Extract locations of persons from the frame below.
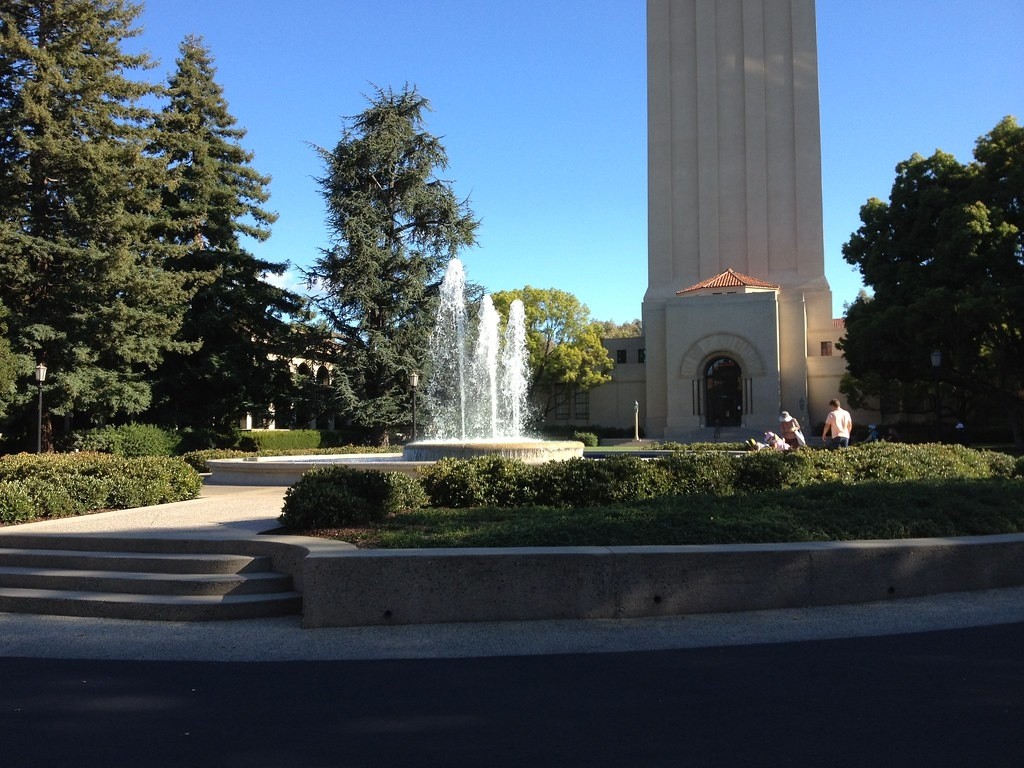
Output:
[747,432,790,453]
[778,411,806,450]
[822,399,852,448]
[887,427,898,442]
[864,425,879,443]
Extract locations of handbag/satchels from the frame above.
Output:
[792,418,806,446]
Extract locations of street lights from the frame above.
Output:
[633,400,642,442]
[33,362,48,456]
[408,372,419,441]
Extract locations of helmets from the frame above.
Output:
[868,425,876,430]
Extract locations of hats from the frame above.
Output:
[779,411,792,422]
